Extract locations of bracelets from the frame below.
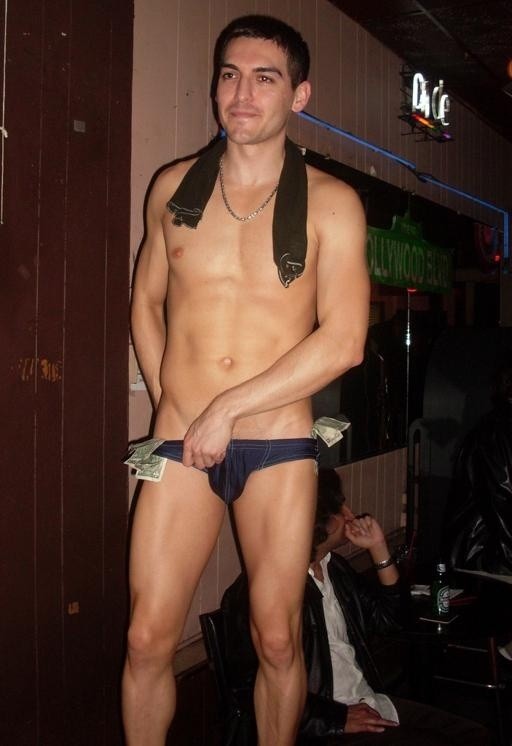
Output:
[374,557,396,571]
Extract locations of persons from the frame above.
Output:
[112,10,372,746]
[216,457,505,745]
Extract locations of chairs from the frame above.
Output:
[198,601,250,743]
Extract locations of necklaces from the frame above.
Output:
[217,151,281,222]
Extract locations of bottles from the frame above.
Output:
[433,557,450,615]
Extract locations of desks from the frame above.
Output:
[369,572,510,741]
